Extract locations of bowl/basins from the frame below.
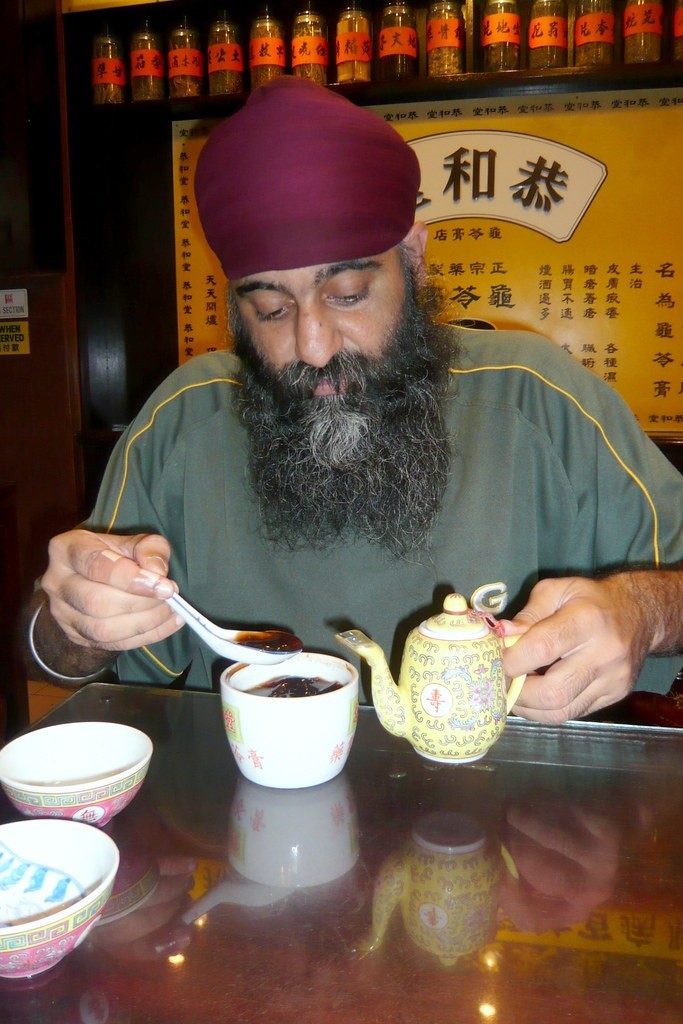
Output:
[0,818,120,979]
[226,775,361,889]
[97,816,158,927]
[0,722,153,831]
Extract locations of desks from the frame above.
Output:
[0,681,683,1024]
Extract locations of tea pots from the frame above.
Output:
[358,814,515,969]
[335,581,530,764]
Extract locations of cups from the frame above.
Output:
[220,653,360,790]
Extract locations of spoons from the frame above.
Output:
[182,879,287,926]
[157,586,301,664]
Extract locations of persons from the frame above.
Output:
[77,784,683,945]
[16,78,682,727]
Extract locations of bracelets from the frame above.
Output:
[28,599,117,685]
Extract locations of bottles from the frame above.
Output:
[92,0,683,106]
